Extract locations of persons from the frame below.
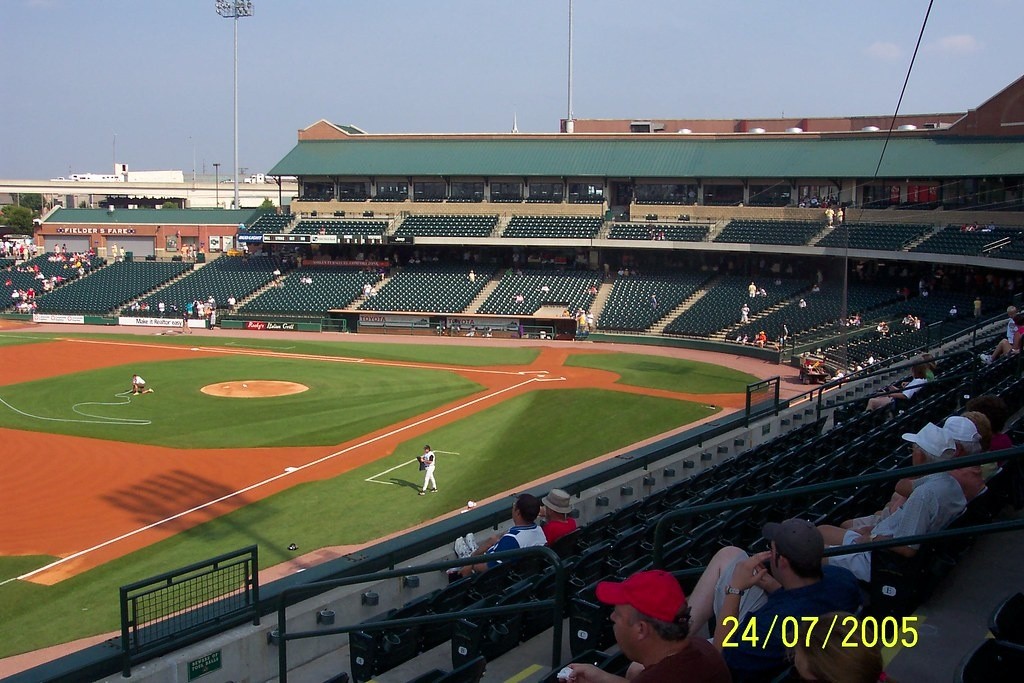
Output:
[447,489,577,576]
[832,354,875,381]
[273,226,386,301]
[888,354,936,395]
[0,221,249,333]
[504,251,608,338]
[409,253,493,337]
[867,364,927,410]
[562,397,1013,683]
[647,223,665,240]
[959,221,999,234]
[417,445,438,495]
[618,267,657,310]
[132,374,154,395]
[796,195,843,228]
[734,266,1024,364]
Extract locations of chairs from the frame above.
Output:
[392,217,1023,654]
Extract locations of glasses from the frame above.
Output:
[765,542,780,556]
[907,443,921,454]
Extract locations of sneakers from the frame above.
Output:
[454,533,480,559]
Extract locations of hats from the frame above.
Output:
[596,570,693,624]
[762,519,824,566]
[541,489,573,513]
[759,331,764,334]
[422,445,429,449]
[800,299,804,302]
[943,416,980,442]
[901,421,956,459]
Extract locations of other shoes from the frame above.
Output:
[826,224,834,228]
[133,392,139,396]
[429,487,438,493]
[418,491,425,496]
[980,353,993,365]
[149,388,154,393]
